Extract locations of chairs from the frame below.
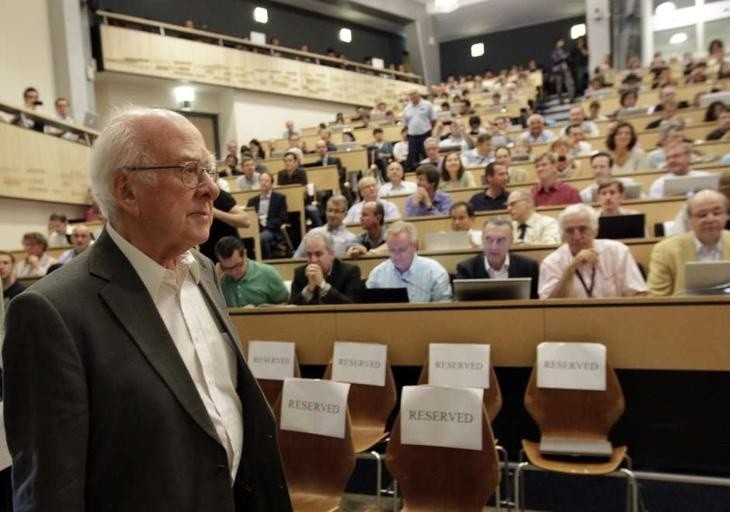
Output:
[387,341,510,512]
[244,339,301,412]
[514,341,637,512]
[271,377,355,512]
[323,342,397,512]
[385,385,503,512]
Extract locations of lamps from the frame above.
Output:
[173,82,193,102]
[655,0,675,19]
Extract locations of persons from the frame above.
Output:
[54,224,96,264]
[202,184,249,263]
[237,160,271,189]
[558,107,600,140]
[591,182,653,238]
[370,128,394,181]
[650,141,716,198]
[653,84,690,112]
[588,101,608,120]
[433,56,543,117]
[539,205,647,297]
[294,194,358,260]
[645,101,687,130]
[545,141,581,176]
[342,201,391,256]
[0,251,29,307]
[214,237,290,308]
[382,160,416,196]
[514,114,556,147]
[401,163,453,219]
[704,101,726,120]
[284,107,366,155]
[586,39,729,92]
[278,151,322,227]
[247,169,289,260]
[648,189,730,296]
[16,87,46,129]
[177,17,427,78]
[717,110,729,127]
[468,116,514,148]
[461,133,497,167]
[0,109,296,512]
[580,152,640,203]
[647,126,696,168]
[605,123,646,172]
[706,116,730,142]
[402,88,436,158]
[456,217,538,298]
[15,233,58,278]
[608,91,638,119]
[437,153,476,187]
[289,232,366,303]
[223,139,286,161]
[394,127,409,171]
[435,108,604,149]
[575,35,589,100]
[221,155,241,179]
[421,138,445,167]
[432,115,477,152]
[50,211,72,248]
[466,159,512,211]
[344,175,401,225]
[476,142,535,188]
[501,189,563,245]
[312,140,353,225]
[440,200,483,249]
[48,93,80,138]
[357,91,414,129]
[530,152,582,206]
[553,40,574,105]
[366,221,455,303]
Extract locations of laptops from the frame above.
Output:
[662,175,720,197]
[699,92,730,108]
[597,214,644,239]
[684,261,729,294]
[451,278,532,301]
[591,185,641,202]
[352,289,410,303]
[423,232,472,253]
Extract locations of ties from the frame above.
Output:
[518,223,529,239]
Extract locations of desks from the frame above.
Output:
[504,102,709,134]
[216,239,670,283]
[224,165,342,222]
[377,164,730,214]
[223,295,730,373]
[581,79,730,115]
[405,140,730,182]
[228,181,306,239]
[17,275,43,288]
[261,124,406,155]
[445,122,725,148]
[81,209,261,264]
[3,246,75,261]
[286,197,686,237]
[368,68,505,120]
[502,66,550,115]
[217,148,368,179]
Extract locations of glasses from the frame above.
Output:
[327,208,345,213]
[363,184,377,189]
[667,152,686,159]
[220,256,244,271]
[507,198,529,206]
[123,162,219,188]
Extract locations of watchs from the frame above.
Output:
[316,277,331,293]
